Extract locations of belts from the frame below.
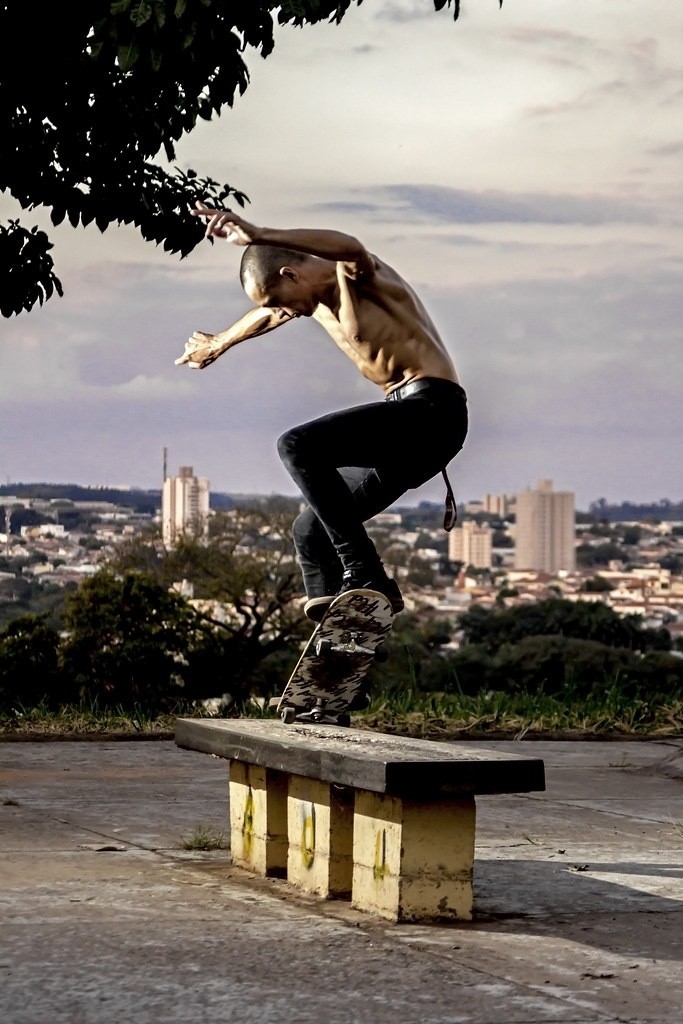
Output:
[385,378,467,401]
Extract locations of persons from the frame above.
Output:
[173,200,469,711]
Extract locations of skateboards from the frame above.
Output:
[277,587,396,726]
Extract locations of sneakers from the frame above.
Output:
[304,570,404,622]
[270,676,371,711]
